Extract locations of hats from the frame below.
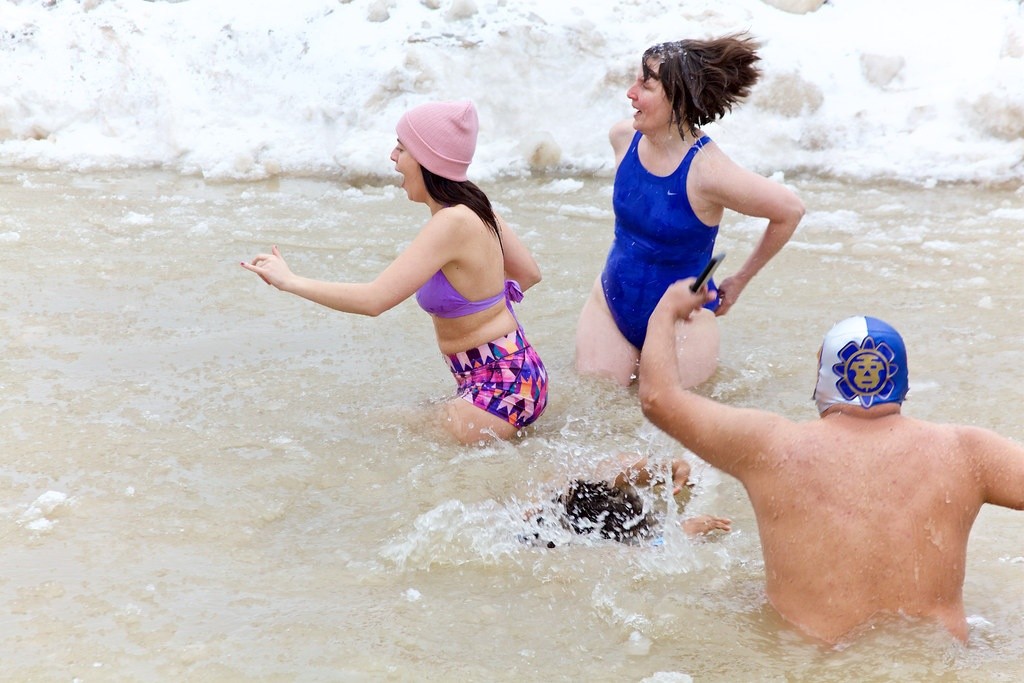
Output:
[813,315,911,411]
[395,103,478,182]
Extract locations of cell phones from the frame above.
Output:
[690,252,726,292]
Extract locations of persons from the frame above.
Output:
[239,97,551,447]
[571,29,809,390]
[520,453,733,550]
[636,274,1023,649]
